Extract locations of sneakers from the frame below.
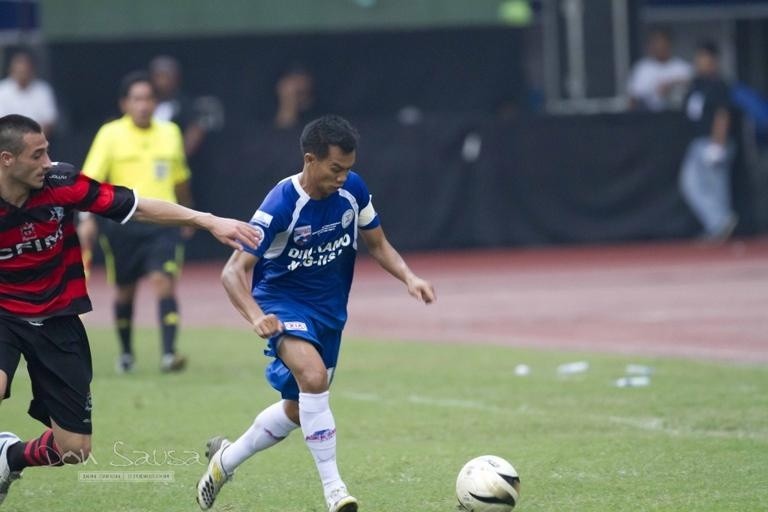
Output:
[115,351,186,375]
[196,436,233,512]
[0,431,25,504]
[328,497,359,512]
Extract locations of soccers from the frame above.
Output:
[455,455,520,512]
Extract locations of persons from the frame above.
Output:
[679,34,747,247]
[0,113,264,505]
[258,69,313,158]
[623,22,688,114]
[147,53,225,162]
[193,114,436,512]
[76,68,198,372]
[1,42,62,141]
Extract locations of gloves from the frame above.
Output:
[702,143,727,169]
